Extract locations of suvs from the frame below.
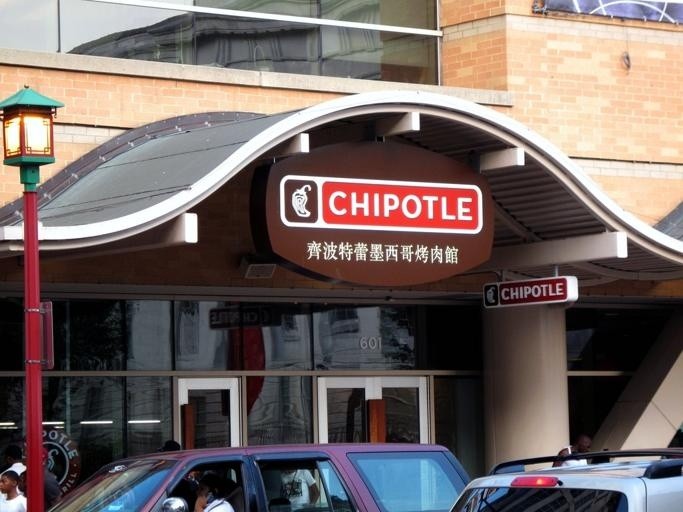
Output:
[448,446,681,510]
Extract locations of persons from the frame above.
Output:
[192,465,238,512]
[278,468,321,511]
[0,444,27,477]
[21,448,62,512]
[551,432,595,469]
[0,470,27,512]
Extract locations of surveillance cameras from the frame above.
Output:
[375,134,386,142]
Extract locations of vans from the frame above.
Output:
[46,443,472,512]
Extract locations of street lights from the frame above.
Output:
[0,86,64,512]
